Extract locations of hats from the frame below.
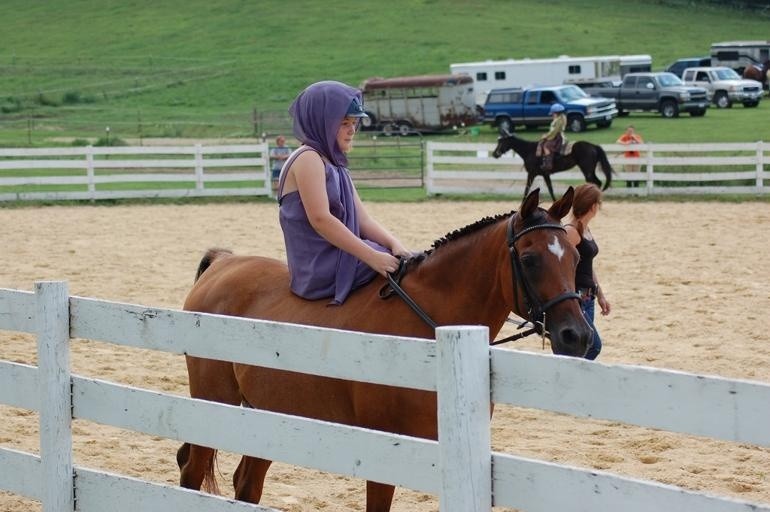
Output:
[344,95,369,121]
[547,102,566,115]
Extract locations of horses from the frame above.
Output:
[491,127,618,207]
[175,185,597,512]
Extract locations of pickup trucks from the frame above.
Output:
[482,57,765,137]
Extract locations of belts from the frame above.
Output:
[579,286,594,297]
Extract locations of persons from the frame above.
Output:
[616,127,646,188]
[540,104,568,175]
[278,81,416,307]
[267,134,293,191]
